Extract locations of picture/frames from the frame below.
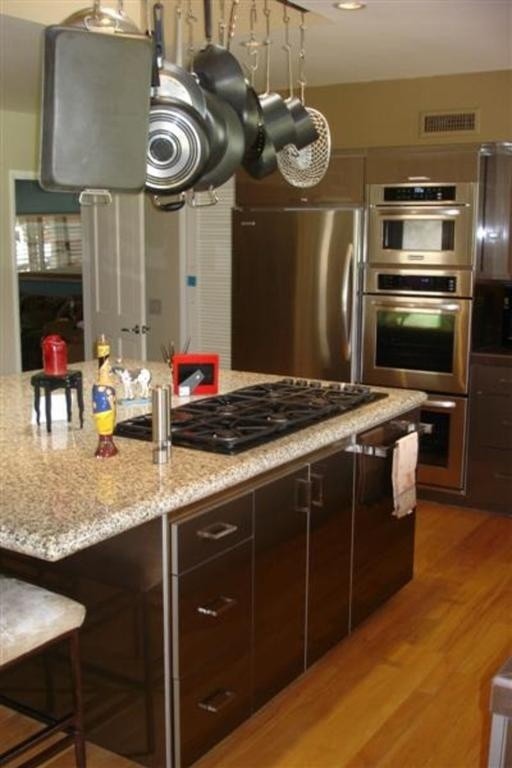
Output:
[170,352,219,398]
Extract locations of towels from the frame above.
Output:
[391,433,418,520]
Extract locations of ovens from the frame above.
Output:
[360,180,477,495]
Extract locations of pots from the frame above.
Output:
[60,0,320,213]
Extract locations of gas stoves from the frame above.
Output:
[114,376,390,457]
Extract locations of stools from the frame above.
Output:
[0,572,89,765]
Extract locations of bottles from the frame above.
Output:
[91,332,120,459]
[147,383,175,455]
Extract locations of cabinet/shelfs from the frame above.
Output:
[355,404,417,631]
[253,441,352,712]
[169,491,255,768]
[469,358,510,511]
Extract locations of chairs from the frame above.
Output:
[16,288,81,372]
